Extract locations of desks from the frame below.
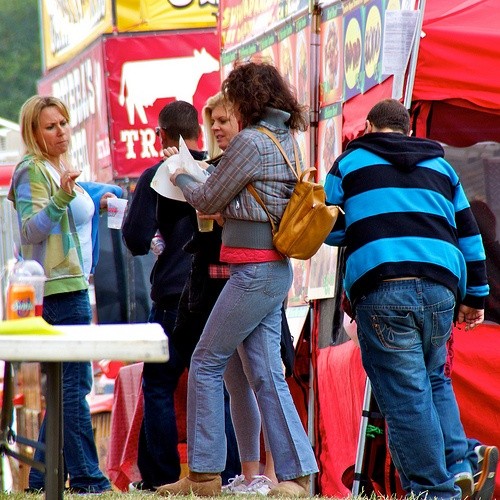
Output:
[0,322,169,500]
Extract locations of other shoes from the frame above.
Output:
[156,469,222,496]
[266,475,310,498]
[128,477,157,491]
[471,445,498,500]
[449,459,474,498]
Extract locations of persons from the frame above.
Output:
[320,99,499,500]
[123,54,320,496]
[7,95,123,493]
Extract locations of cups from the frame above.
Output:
[152,232,165,255]
[195,209,214,233]
[106,197,128,229]
[8,260,47,316]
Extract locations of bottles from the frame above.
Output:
[6,267,36,320]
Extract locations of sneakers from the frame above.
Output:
[232,474,274,496]
[222,475,251,493]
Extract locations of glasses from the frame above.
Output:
[155,127,166,135]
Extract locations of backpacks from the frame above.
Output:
[244,128,344,260]
